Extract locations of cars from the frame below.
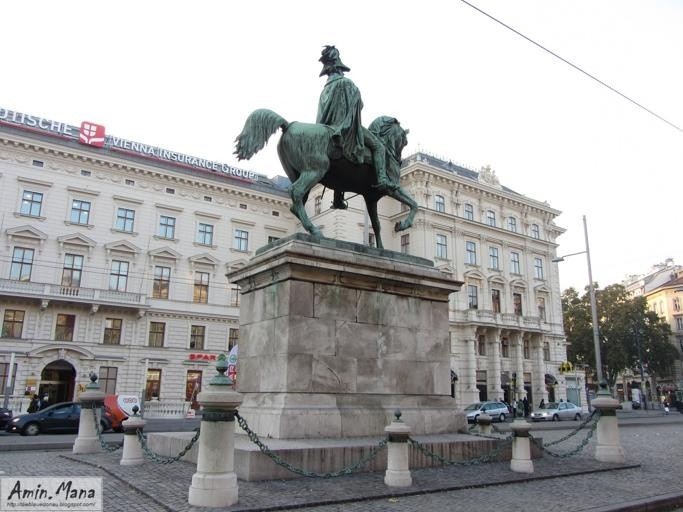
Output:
[462,400,509,423]
[616,401,639,410]
[0,408,13,428]
[529,401,582,422]
[5,402,111,435]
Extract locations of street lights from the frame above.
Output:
[550,215,604,394]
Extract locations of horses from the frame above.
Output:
[232,108,418,251]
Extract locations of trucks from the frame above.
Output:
[102,394,141,432]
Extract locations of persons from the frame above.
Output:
[28,394,38,413]
[540,399,544,409]
[318,46,400,208]
[499,397,529,418]
[21,391,32,413]
[40,395,49,410]
[663,399,669,416]
